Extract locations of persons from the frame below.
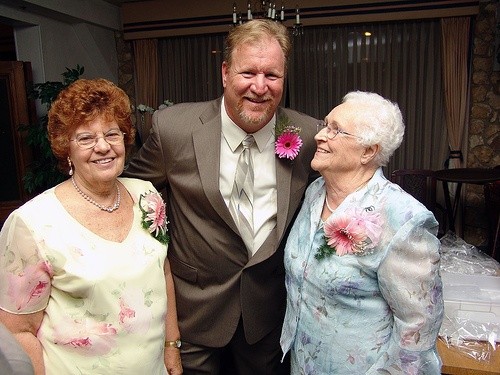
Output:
[119,19,330,375]
[280,90,443,375]
[0,76,183,375]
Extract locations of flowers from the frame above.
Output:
[132,97,175,112]
[314,203,384,260]
[139,190,169,244]
[271,113,303,160]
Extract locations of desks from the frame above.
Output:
[433,168,500,258]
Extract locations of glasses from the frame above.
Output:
[68,128,126,150]
[316,120,361,140]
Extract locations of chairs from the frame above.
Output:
[391,169,446,238]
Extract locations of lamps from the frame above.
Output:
[233,0,304,36]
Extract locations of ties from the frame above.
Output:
[228,135,255,261]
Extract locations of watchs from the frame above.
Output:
[164,337,182,349]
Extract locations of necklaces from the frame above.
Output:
[71,172,122,212]
